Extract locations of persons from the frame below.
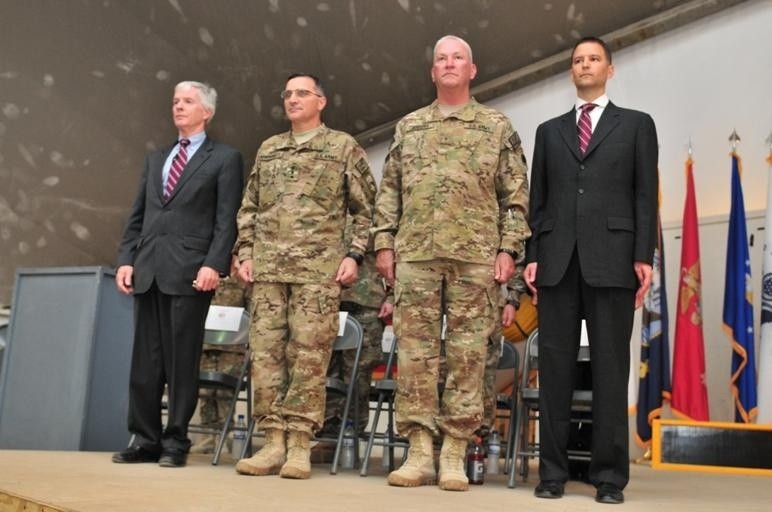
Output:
[190,268,254,454]
[521,37,659,503]
[232,75,377,480]
[370,35,532,492]
[112,81,243,467]
[434,266,525,472]
[310,235,395,470]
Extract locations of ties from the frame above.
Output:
[164,139,189,200]
[578,103,597,152]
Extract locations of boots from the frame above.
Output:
[281,430,314,479]
[439,433,469,491]
[309,441,336,464]
[235,427,287,476]
[188,430,217,454]
[216,432,231,457]
[387,430,437,488]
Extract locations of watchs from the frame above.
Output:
[344,252,363,266]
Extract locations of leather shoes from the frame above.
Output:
[159,446,187,467]
[596,482,624,505]
[533,478,566,498]
[112,438,165,463]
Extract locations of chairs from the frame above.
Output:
[509,324,592,488]
[361,329,520,483]
[162,303,255,464]
[240,311,359,472]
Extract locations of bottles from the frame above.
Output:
[230,413,248,460]
[379,425,397,466]
[486,429,502,476]
[466,435,485,486]
[342,419,356,470]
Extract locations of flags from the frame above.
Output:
[634,183,671,450]
[757,155,772,424]
[671,158,709,422]
[722,151,759,422]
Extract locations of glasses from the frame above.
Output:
[280,90,321,98]
